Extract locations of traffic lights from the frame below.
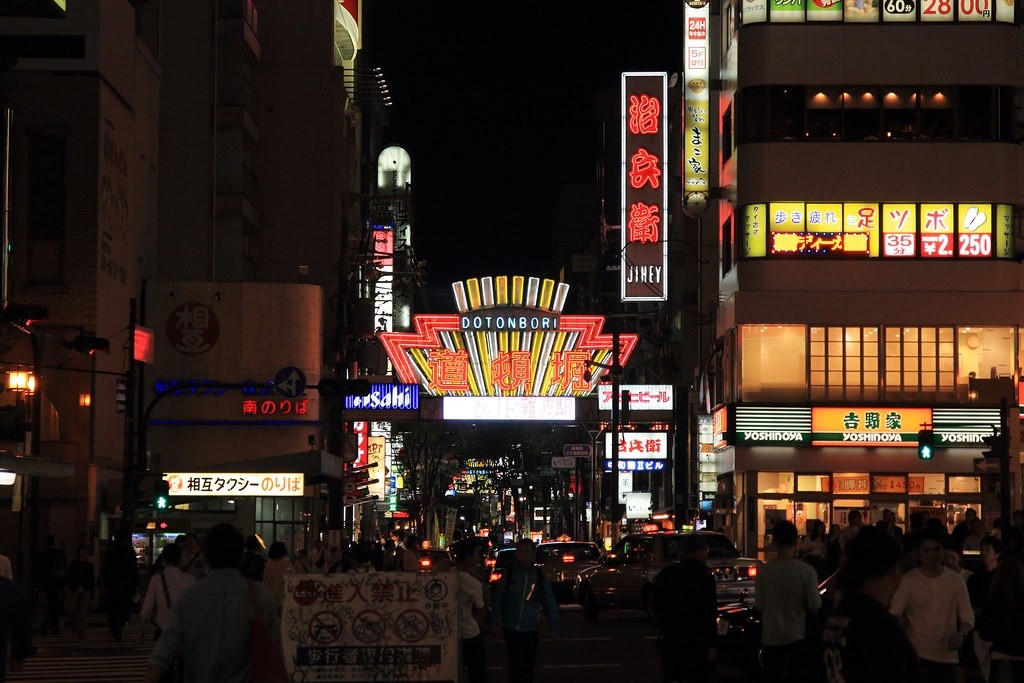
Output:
[154,480,169,511]
[74,334,111,356]
[918,430,935,459]
[158,522,168,528]
[982,437,1001,462]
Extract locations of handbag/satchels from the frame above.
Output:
[248,577,290,683]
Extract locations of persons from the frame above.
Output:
[755,520,822,682]
[0,523,491,683]
[801,508,1024,682]
[491,538,560,683]
[651,533,718,682]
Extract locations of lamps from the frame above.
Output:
[299,266,310,283]
[214,293,220,301]
[309,435,315,451]
[414,261,427,288]
[169,292,176,300]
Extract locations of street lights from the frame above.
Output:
[582,363,619,553]
[6,364,39,580]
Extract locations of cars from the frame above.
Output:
[418,538,609,603]
[571,530,763,626]
